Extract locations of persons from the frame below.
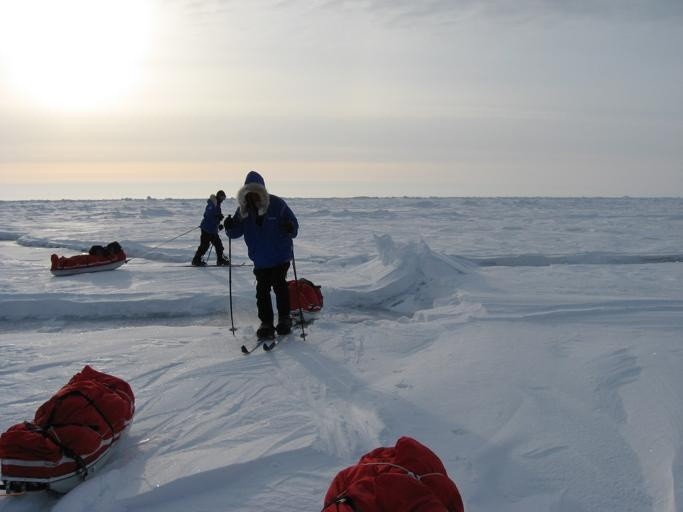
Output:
[223,171,297,339]
[189,190,229,265]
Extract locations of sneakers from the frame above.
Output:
[191,257,230,266]
[254,317,291,338]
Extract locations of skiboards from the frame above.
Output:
[165,262,255,267]
[242,326,298,354]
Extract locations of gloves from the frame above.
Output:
[223,214,234,230]
[281,218,294,234]
